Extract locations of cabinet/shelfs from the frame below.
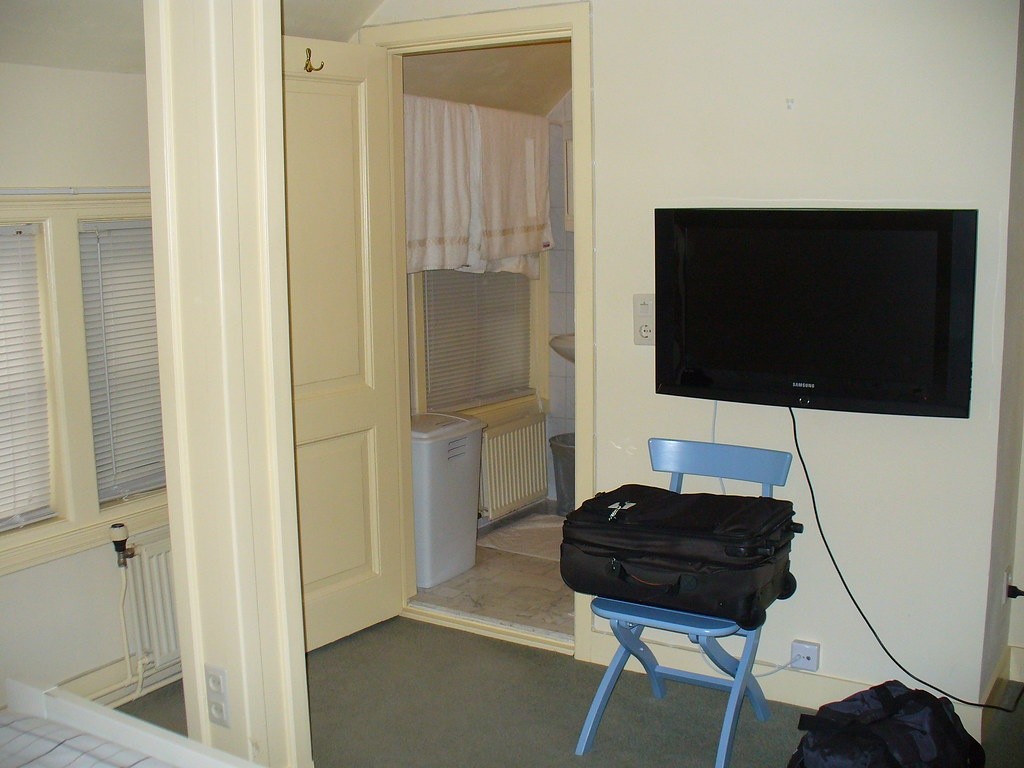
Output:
[457,394,551,520]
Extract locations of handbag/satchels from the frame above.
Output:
[792,677,985,768]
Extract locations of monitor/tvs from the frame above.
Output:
[655,207,979,420]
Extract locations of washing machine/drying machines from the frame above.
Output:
[410,410,488,589]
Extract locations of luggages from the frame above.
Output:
[562,482,802,630]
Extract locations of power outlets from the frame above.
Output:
[632,294,655,347]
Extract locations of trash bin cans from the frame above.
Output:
[549,433,575,517]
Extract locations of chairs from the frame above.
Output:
[569,436,794,768]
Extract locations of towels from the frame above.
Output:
[404,92,556,280]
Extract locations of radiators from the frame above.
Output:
[126,539,184,674]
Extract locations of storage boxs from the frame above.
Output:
[411,410,490,590]
